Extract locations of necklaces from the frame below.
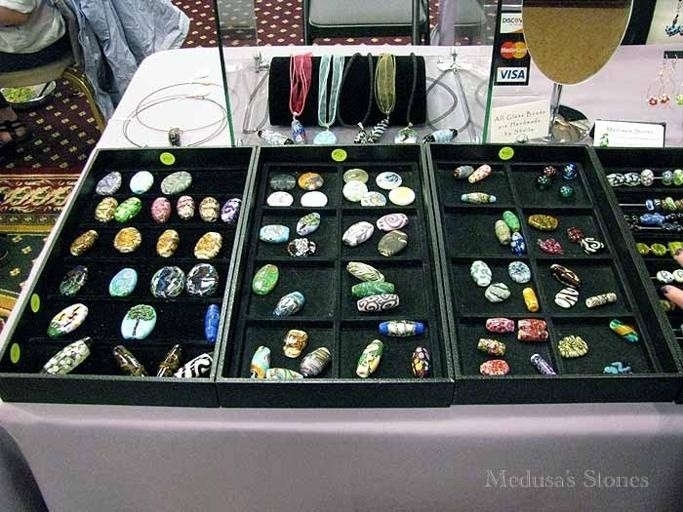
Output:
[241,50,471,148]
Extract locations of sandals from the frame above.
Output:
[0,118,30,148]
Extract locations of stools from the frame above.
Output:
[0,55,106,139]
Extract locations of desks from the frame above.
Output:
[0,46,682,511]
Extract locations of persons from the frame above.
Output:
[0,0,70,150]
[660,247,682,312]
